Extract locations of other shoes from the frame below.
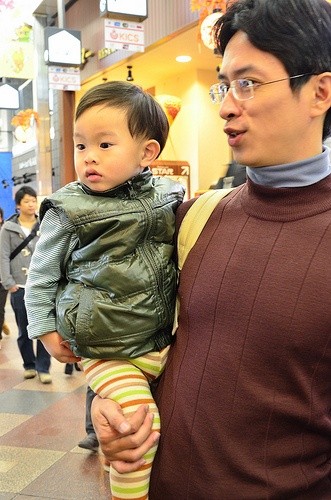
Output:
[3,324,9,335]
[23,369,36,378]
[74,362,81,371]
[65,363,73,374]
[39,373,52,383]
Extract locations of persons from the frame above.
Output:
[23,0,330,500]
[0,187,85,384]
[25,80,186,500]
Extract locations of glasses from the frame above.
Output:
[208,73,319,104]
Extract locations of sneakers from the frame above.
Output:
[78,433,99,452]
